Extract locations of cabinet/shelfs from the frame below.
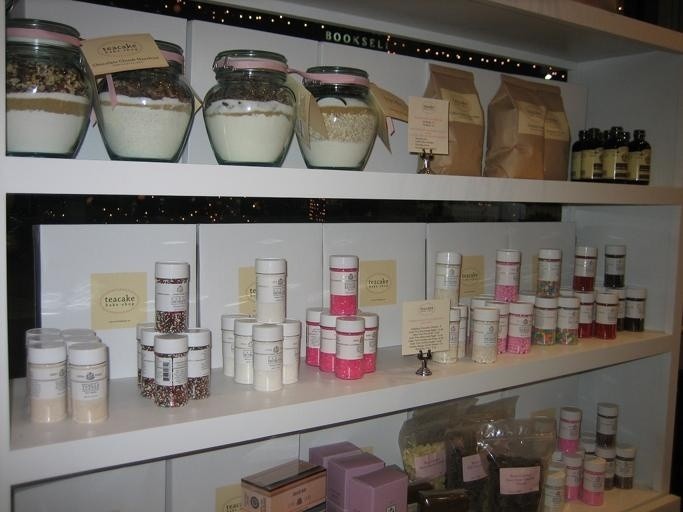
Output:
[1,0,683,512]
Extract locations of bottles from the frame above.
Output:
[495,247,519,303]
[27,325,111,423]
[93,39,196,163]
[532,292,647,348]
[328,255,360,316]
[543,432,635,512]
[256,258,289,324]
[596,401,619,449]
[220,313,302,392]
[433,251,463,310]
[304,308,380,381]
[6,15,93,156]
[602,244,626,290]
[152,262,189,333]
[201,50,299,166]
[561,406,583,454]
[431,292,536,364]
[536,247,561,298]
[572,245,597,292]
[136,321,212,410]
[298,67,376,170]
[572,127,654,185]
